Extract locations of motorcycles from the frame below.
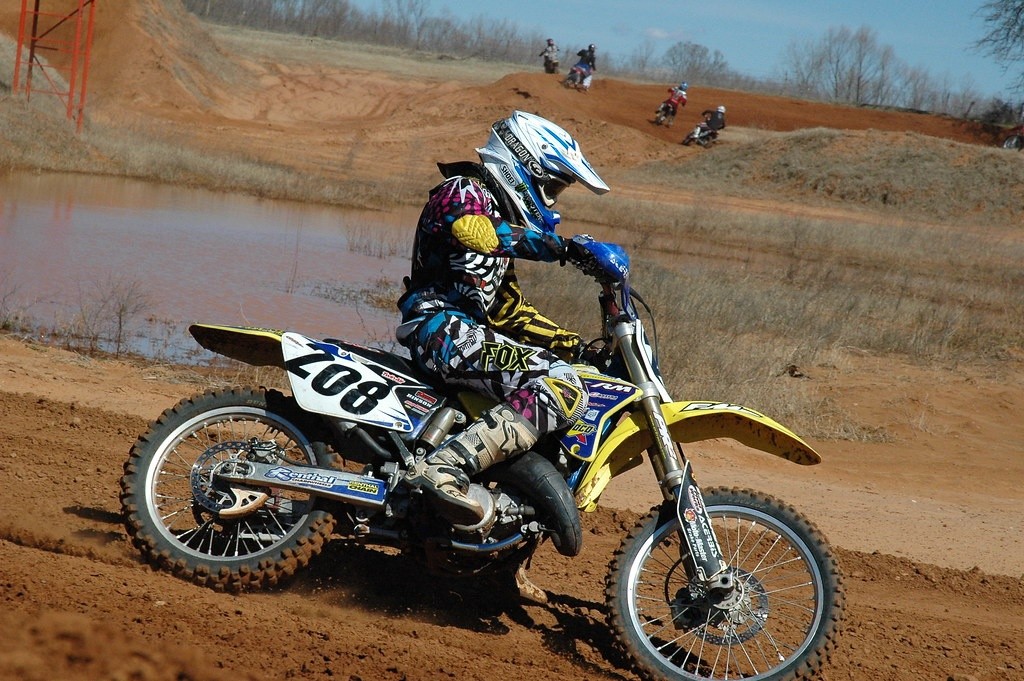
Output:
[565,64,588,88]
[119,248,846,681]
[657,103,674,124]
[681,116,718,148]
[545,60,561,76]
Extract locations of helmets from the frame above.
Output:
[680,83,687,91]
[717,105,726,114]
[589,44,596,50]
[547,38,553,44]
[474,110,610,234]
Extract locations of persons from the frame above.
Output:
[656,79,687,124]
[691,105,725,138]
[559,43,598,89]
[540,37,561,67]
[398,110,629,605]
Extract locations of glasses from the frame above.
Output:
[536,172,569,207]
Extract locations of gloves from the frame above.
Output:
[565,232,605,273]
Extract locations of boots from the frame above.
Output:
[488,548,549,604]
[387,403,540,524]
